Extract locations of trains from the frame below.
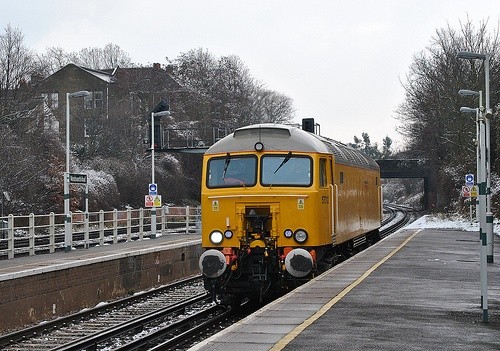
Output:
[198,118,384,304]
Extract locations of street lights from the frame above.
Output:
[459,104,481,222]
[63,89,91,255]
[150,111,171,236]
[456,49,495,263]
[457,89,487,253]
